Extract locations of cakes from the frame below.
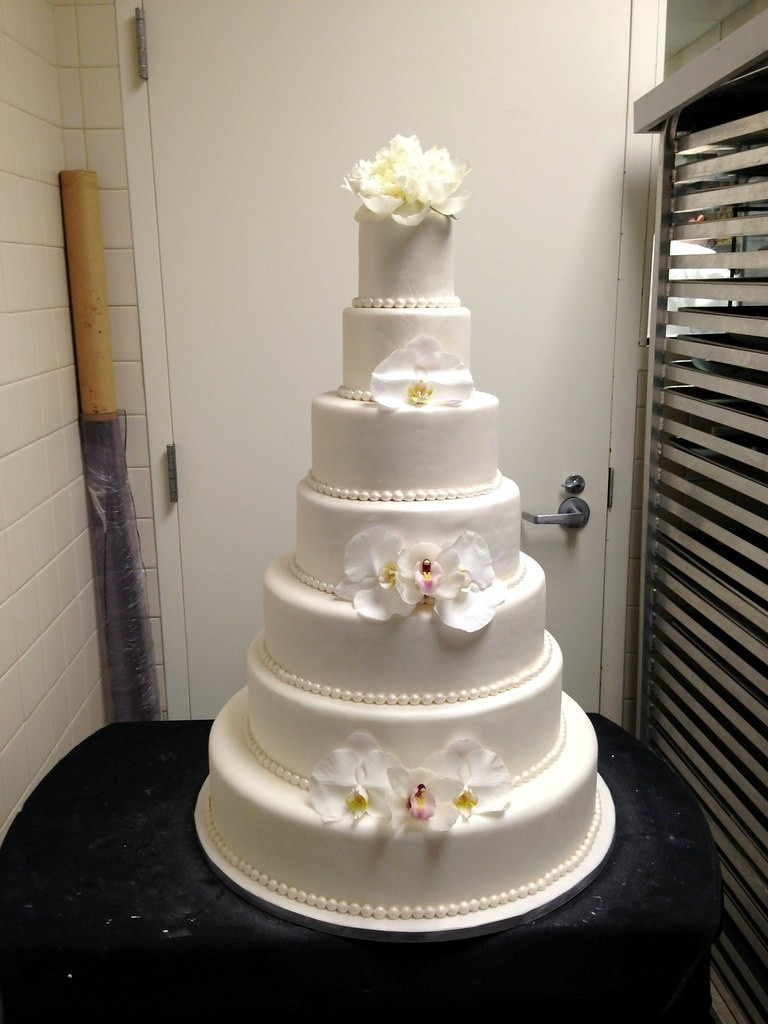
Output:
[205,132,601,921]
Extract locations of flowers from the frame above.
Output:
[338,131,472,226]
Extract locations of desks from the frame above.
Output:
[0,711,727,1024]
